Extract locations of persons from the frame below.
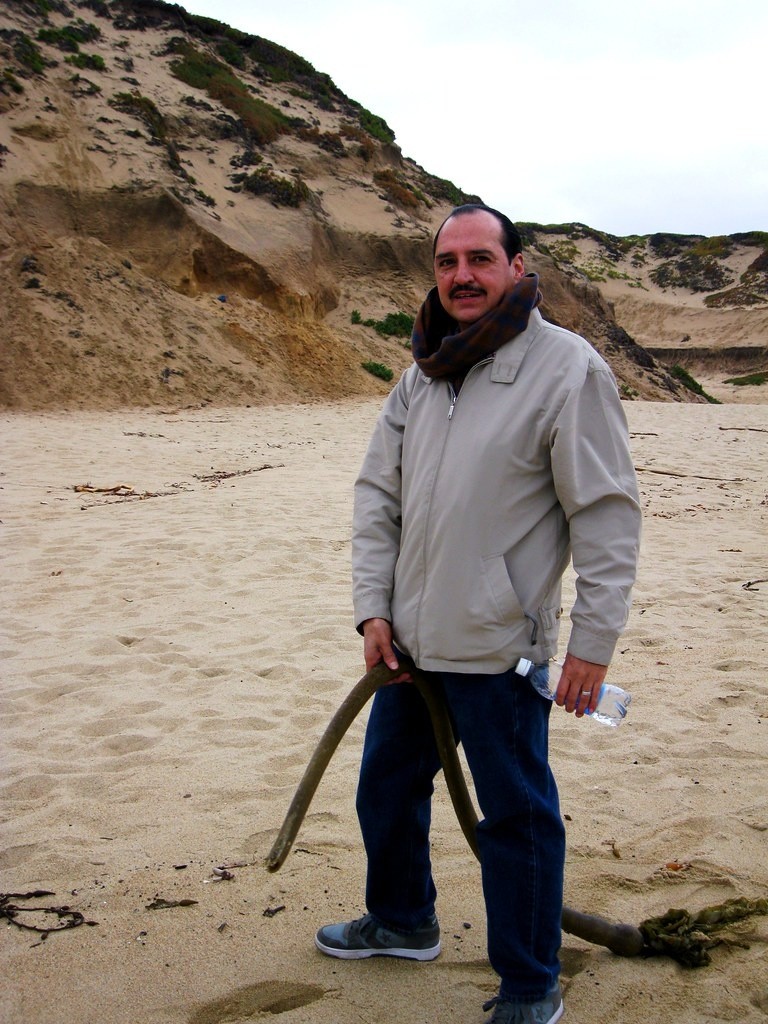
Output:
[316,203,642,1024]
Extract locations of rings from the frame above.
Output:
[581,690,592,697]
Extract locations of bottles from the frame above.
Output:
[514,658,631,727]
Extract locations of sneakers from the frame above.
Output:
[314,912,440,961]
[483,988,563,1024]
